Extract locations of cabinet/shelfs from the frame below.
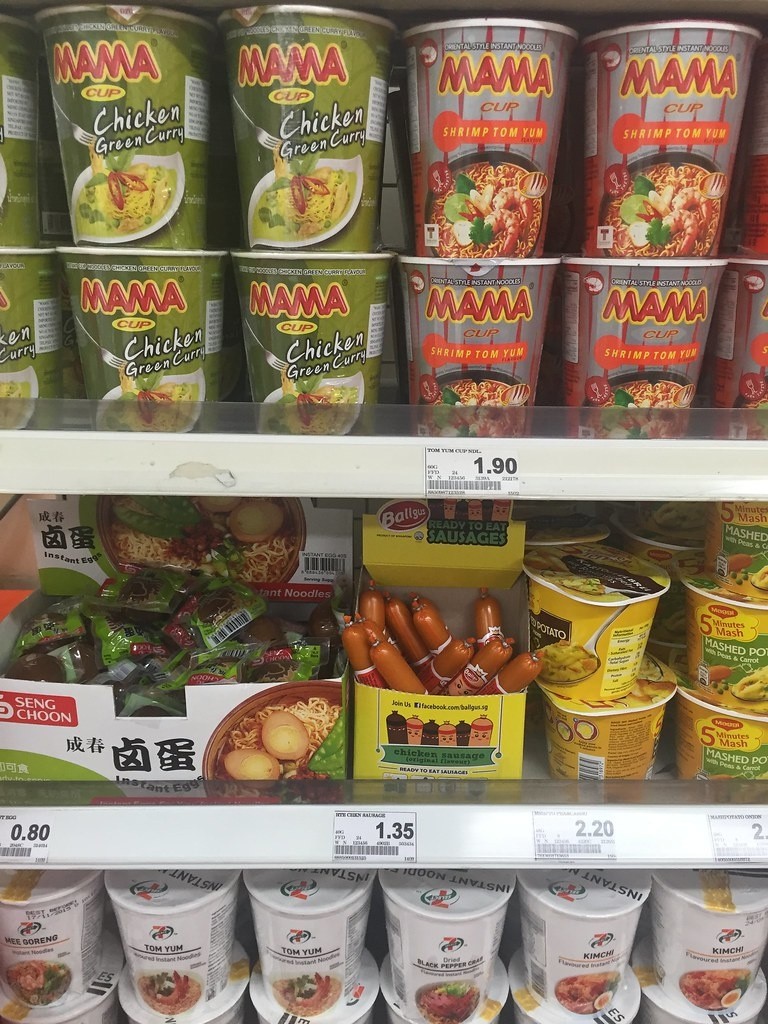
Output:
[2,394,766,873]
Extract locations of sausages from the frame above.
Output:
[341,577,544,695]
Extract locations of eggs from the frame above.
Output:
[4,567,350,717]
[199,495,285,542]
[223,711,310,789]
